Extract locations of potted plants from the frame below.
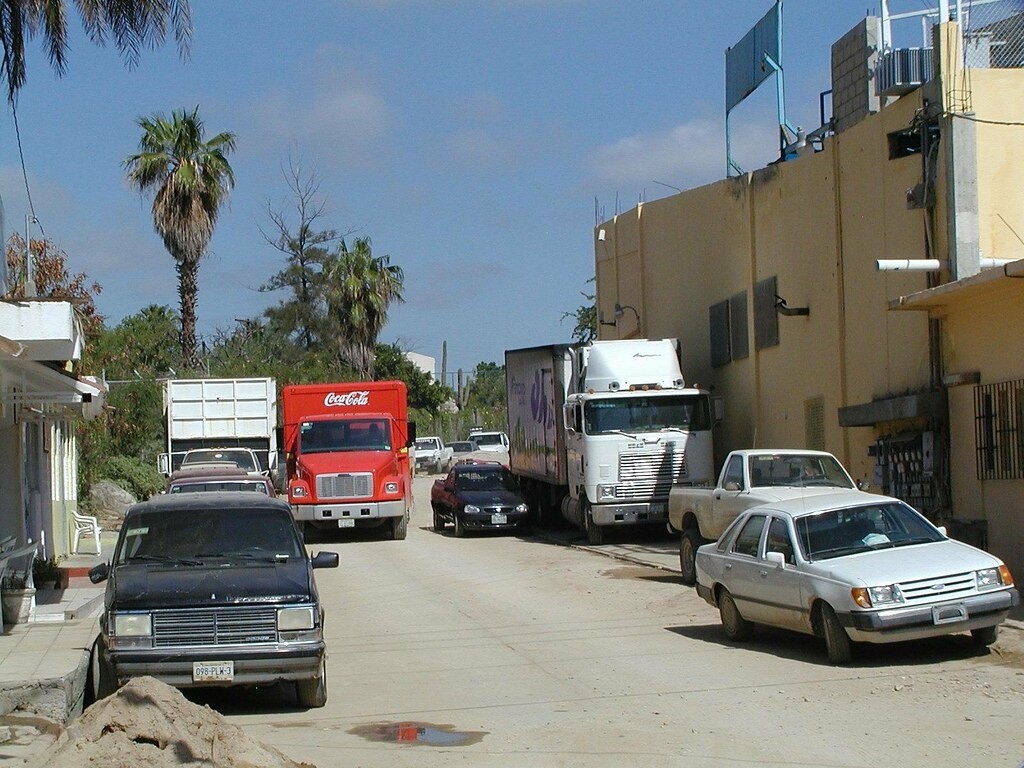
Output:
[2,573,35,624]
[32,552,68,592]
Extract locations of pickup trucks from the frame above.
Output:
[667,449,860,586]
[414,436,454,475]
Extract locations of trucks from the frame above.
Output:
[282,380,411,540]
[157,376,277,498]
[505,338,716,545]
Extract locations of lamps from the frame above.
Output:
[615,303,641,328]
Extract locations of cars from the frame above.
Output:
[430,461,533,539]
[696,490,1020,666]
[88,447,339,709]
[445,431,509,471]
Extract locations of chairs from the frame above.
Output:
[367,423,385,444]
[72,511,103,556]
[791,467,800,481]
[752,468,764,484]
[458,476,479,491]
[481,477,501,489]
[843,518,881,543]
